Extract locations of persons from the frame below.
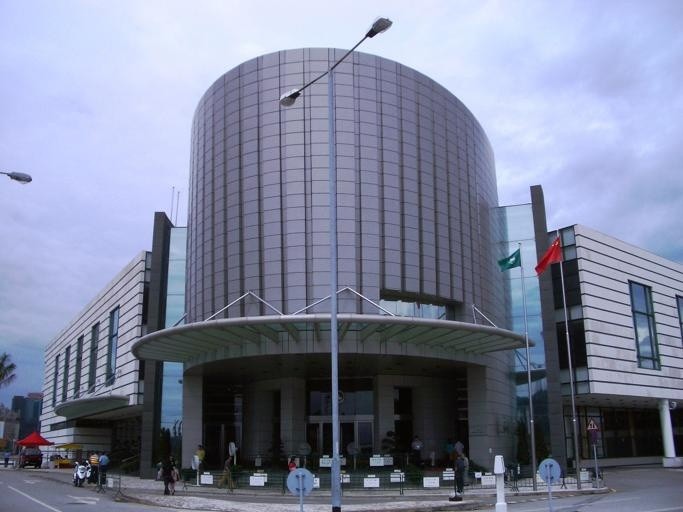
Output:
[4,450,11,467]
[197,444,205,472]
[155,456,180,496]
[447,439,470,491]
[287,456,296,473]
[15,446,21,455]
[217,457,236,489]
[85,448,110,485]
[191,452,202,478]
[381,431,423,466]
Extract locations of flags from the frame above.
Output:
[497,248,521,272]
[535,237,561,275]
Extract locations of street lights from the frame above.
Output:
[279,14,391,510]
[0,171,31,184]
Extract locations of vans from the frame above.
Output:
[19,448,43,469]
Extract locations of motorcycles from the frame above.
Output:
[72,461,94,487]
